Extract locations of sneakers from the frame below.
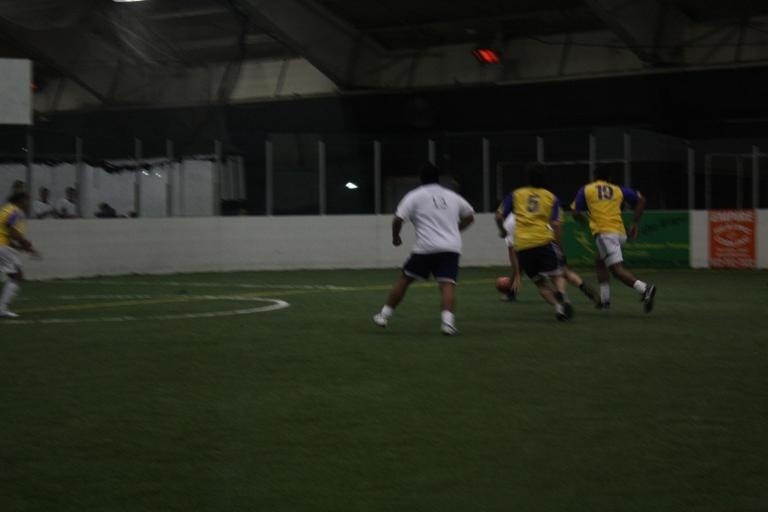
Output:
[551,289,574,322]
[640,282,657,313]
[371,311,391,327]
[439,320,462,335]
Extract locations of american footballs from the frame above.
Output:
[496,278,517,296]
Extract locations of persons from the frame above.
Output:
[0,191,43,319]
[502,211,602,309]
[493,161,575,321]
[568,165,658,314]
[370,162,475,336]
[98,202,115,217]
[33,186,56,219]
[7,178,27,218]
[55,187,79,217]
